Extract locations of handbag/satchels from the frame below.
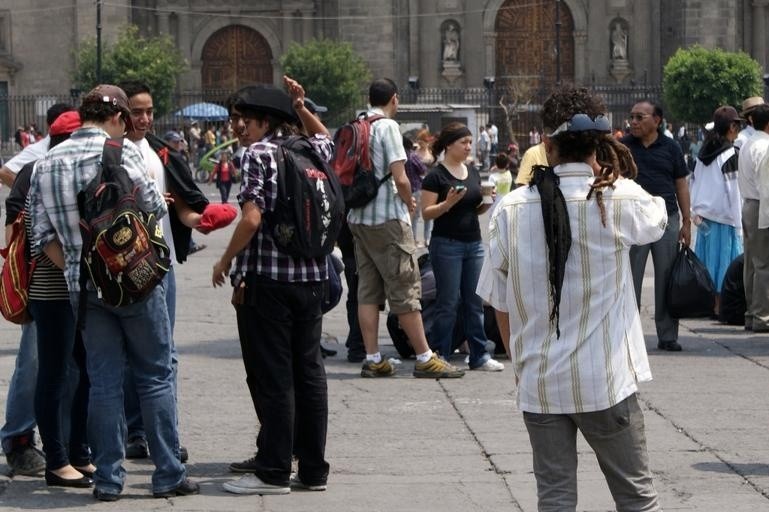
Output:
[0,211,37,324]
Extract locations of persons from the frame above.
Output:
[474,89,669,510]
[1,103,82,189]
[165,116,248,254]
[1,111,83,477]
[22,184,98,487]
[227,75,302,474]
[25,83,200,503]
[285,77,550,381]
[116,83,214,462]
[614,99,768,351]
[213,75,329,493]
[610,21,628,61]
[442,23,461,61]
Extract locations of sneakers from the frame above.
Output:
[658,341,683,351]
[412,351,465,378]
[5,445,199,500]
[474,359,504,371]
[360,354,399,377]
[222,458,326,495]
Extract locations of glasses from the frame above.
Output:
[630,114,651,122]
[546,112,612,137]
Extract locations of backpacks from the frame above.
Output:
[77,139,171,307]
[264,135,346,259]
[331,112,395,209]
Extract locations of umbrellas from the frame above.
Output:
[175,100,233,135]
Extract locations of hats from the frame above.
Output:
[303,97,328,113]
[714,107,746,121]
[49,111,82,136]
[87,84,137,135]
[743,97,765,114]
